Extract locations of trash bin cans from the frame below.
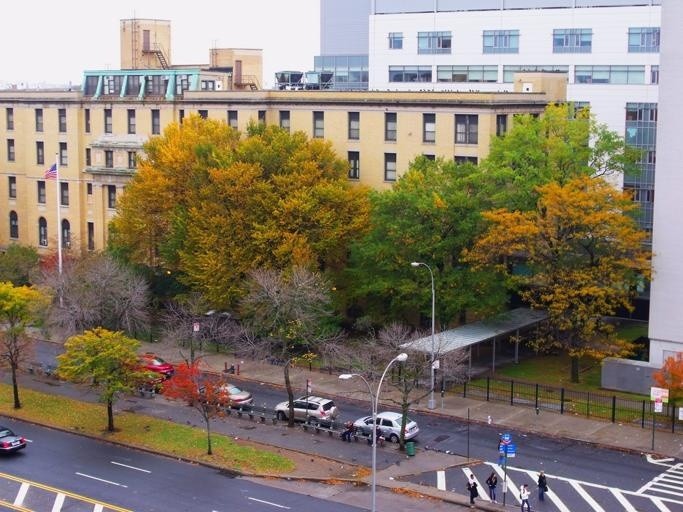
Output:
[407,442,415,456]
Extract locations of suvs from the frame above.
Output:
[274,395,337,427]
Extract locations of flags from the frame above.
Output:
[46,164,57,179]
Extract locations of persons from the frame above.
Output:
[342,421,354,443]
[486,473,498,504]
[467,474,478,504]
[519,483,531,512]
[371,425,382,448]
[538,470,546,502]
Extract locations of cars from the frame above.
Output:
[124,355,254,408]
[353,411,419,443]
[0,425,26,453]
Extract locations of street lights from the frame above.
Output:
[410,261,437,410]
[339,353,408,512]
